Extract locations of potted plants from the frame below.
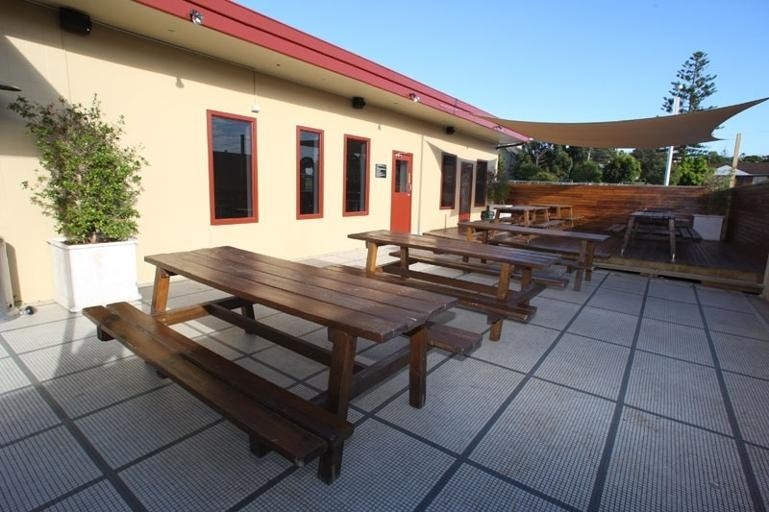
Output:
[10,92,150,315]
[691,150,745,241]
[487,153,513,219]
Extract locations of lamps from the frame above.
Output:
[251,72,261,114]
